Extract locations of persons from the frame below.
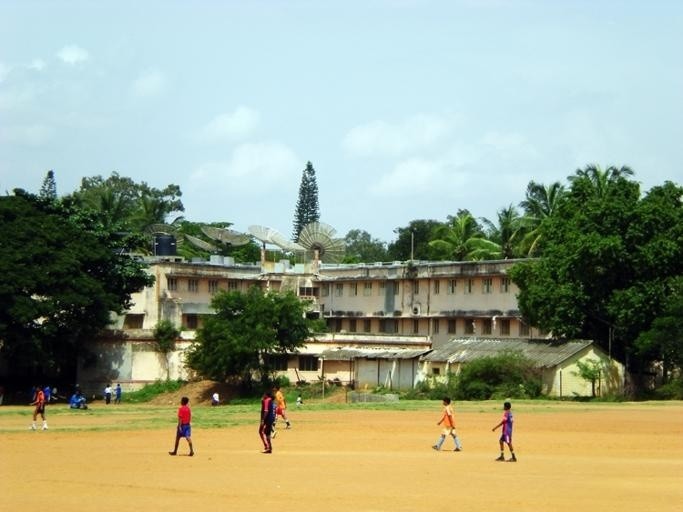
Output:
[29,386,48,430]
[211,390,221,405]
[270,395,277,439]
[260,389,276,454]
[113,385,122,403]
[169,397,195,456]
[104,384,113,402]
[431,397,461,453]
[32,382,66,401]
[296,393,304,404]
[492,401,517,464]
[70,389,88,410]
[272,385,291,429]
